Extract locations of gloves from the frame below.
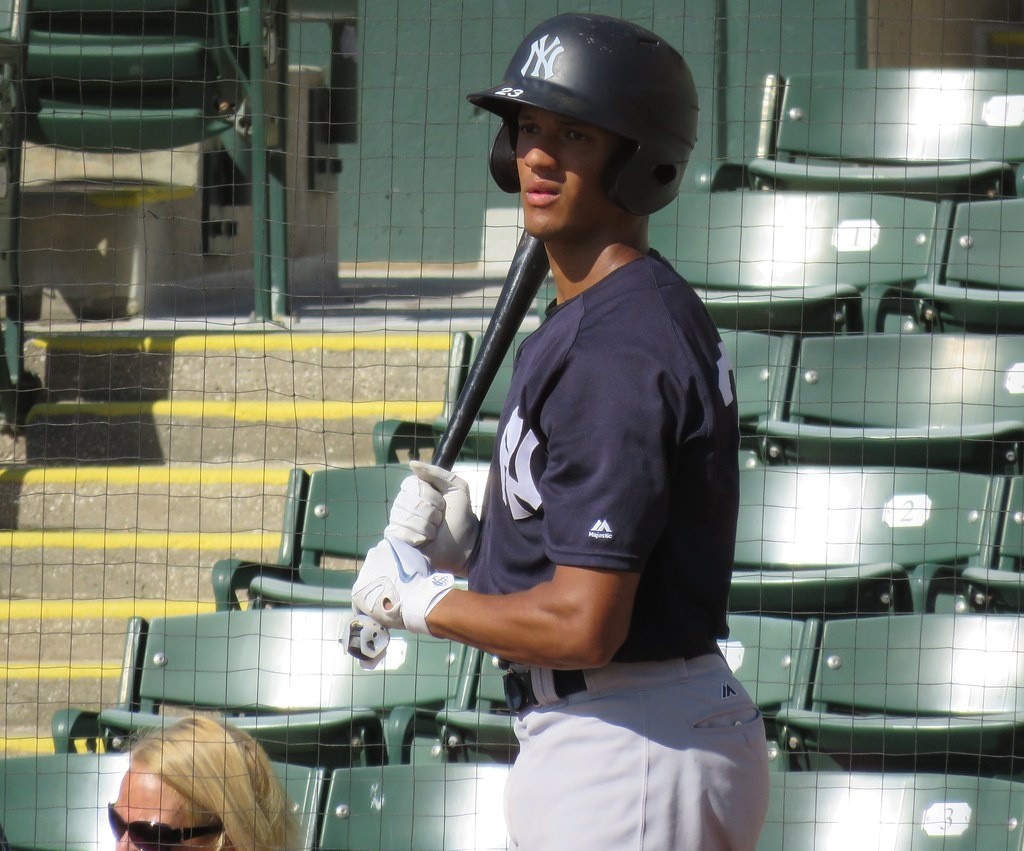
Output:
[351,538,454,641]
[384,459,479,580]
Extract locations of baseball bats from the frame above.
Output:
[347,229,552,662]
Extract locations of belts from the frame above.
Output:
[502,638,722,715]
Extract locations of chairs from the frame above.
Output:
[0,0,1024,851]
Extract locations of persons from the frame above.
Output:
[350,14,768,850]
[107,716,291,851]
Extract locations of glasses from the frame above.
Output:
[108,801,222,851]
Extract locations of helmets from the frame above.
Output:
[465,13,698,216]
